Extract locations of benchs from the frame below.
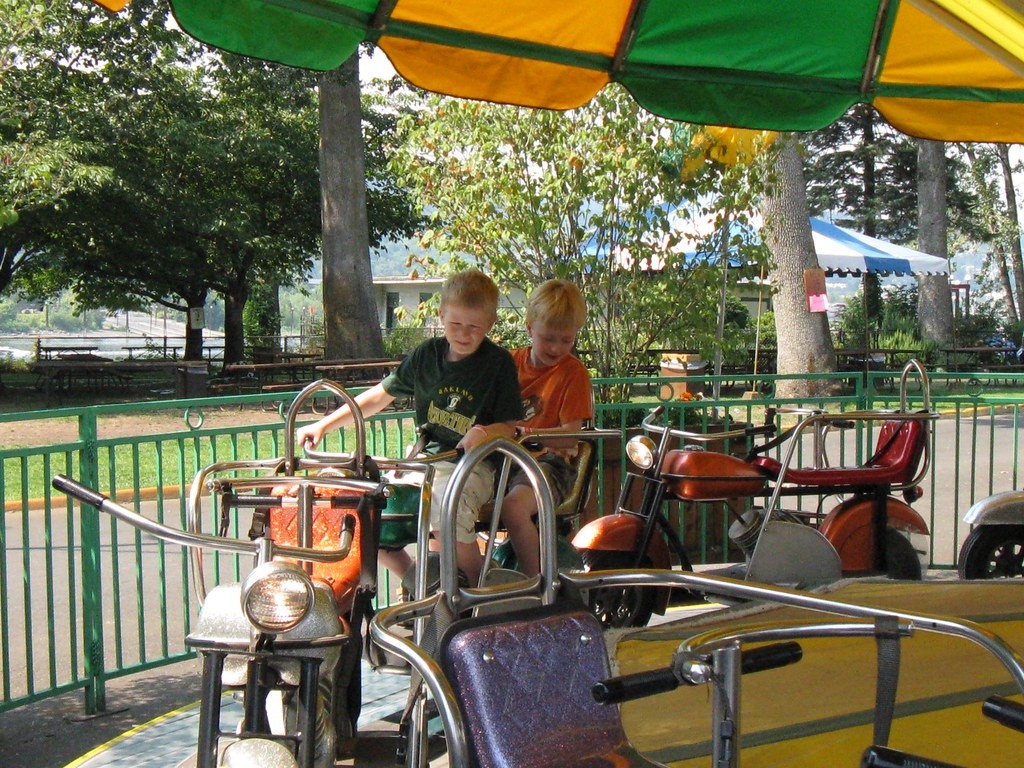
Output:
[27,343,405,398]
[596,345,1024,393]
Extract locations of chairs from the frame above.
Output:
[754,419,921,486]
[438,602,665,768]
[268,484,361,618]
[478,435,599,525]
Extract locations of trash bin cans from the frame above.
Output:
[849,355,886,388]
[253,345,282,375]
[660,353,709,400]
[175,360,209,409]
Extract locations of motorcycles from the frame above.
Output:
[50,374,960,768]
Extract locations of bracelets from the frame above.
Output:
[473,425,488,436]
[526,427,532,434]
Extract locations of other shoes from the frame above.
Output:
[396,585,416,629]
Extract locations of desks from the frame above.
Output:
[835,348,921,371]
[39,346,99,358]
[316,360,404,384]
[940,348,1019,364]
[122,345,183,360]
[203,346,253,359]
[274,353,319,360]
[227,362,316,394]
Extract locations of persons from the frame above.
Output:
[296,269,527,590]
[507,278,593,577]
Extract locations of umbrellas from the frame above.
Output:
[542,189,950,278]
[87,0,1022,143]
